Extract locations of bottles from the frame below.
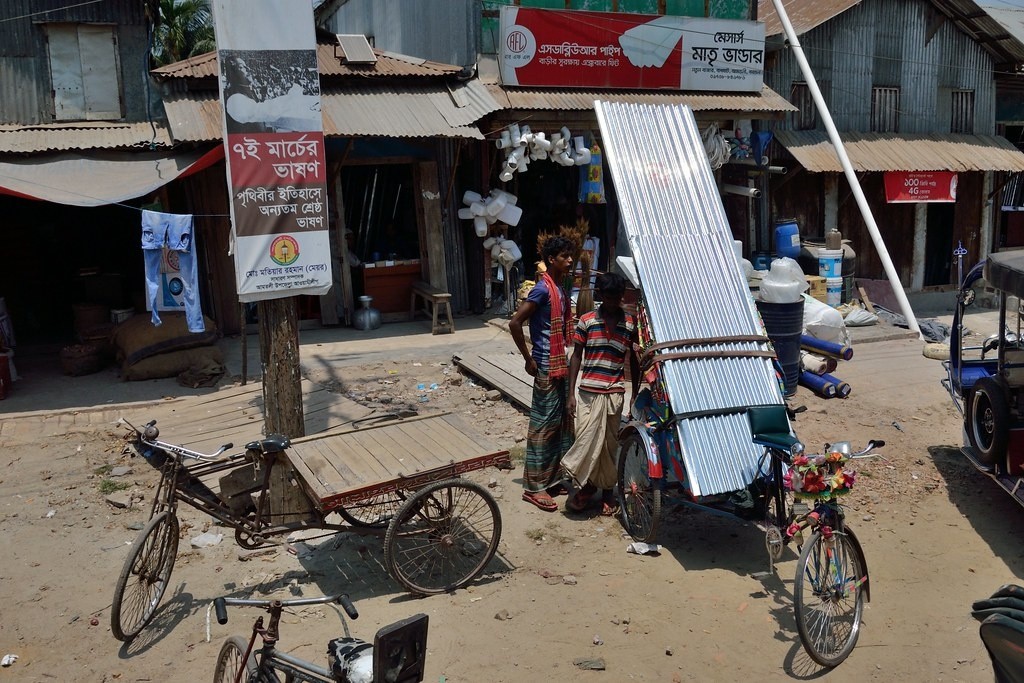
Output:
[483,237,522,272]
[826,228,841,249]
[458,189,522,237]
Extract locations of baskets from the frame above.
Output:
[793,455,856,497]
[59,343,103,370]
[83,327,117,358]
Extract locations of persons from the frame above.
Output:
[508,237,574,511]
[559,272,640,515]
[223,53,320,133]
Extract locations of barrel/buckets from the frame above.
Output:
[819,248,843,277]
[80,306,105,328]
[774,219,800,258]
[827,277,843,308]
[841,258,856,304]
[750,286,805,396]
[110,306,135,325]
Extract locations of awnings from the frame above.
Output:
[0,93,800,206]
[778,133,1024,172]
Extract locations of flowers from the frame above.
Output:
[781,453,855,495]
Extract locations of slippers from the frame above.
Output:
[567,489,597,510]
[522,491,559,509]
[601,492,617,515]
[548,482,568,494]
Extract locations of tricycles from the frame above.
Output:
[941,250,1023,511]
[614,341,891,669]
[109,409,517,643]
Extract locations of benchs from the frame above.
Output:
[409,279,455,335]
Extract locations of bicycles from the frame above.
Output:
[205,591,430,683]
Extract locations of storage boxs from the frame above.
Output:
[362,259,420,268]
[805,275,826,303]
[60,347,102,377]
